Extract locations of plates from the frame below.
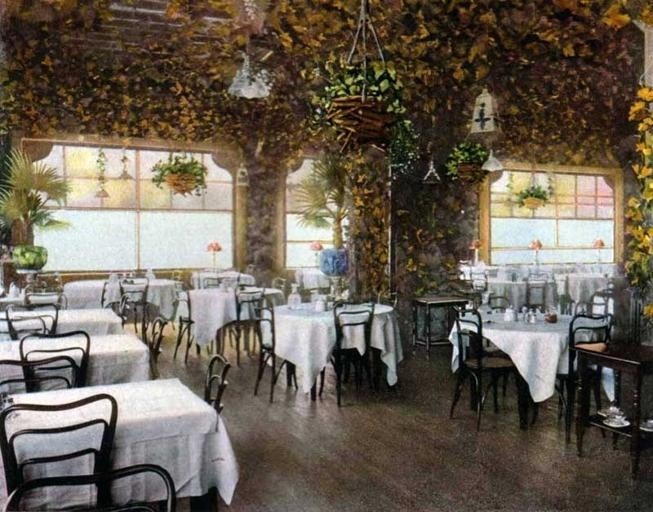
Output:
[639,420,653,431]
[596,405,630,428]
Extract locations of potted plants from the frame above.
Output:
[150,155,208,198]
[294,159,350,276]
[308,60,421,179]
[515,185,549,210]
[444,143,488,186]
[0,145,72,271]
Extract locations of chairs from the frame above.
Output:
[447,262,615,445]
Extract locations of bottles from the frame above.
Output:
[527,307,538,325]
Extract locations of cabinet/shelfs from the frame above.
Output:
[410,296,468,360]
[569,339,652,480]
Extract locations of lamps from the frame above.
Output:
[467,81,503,145]
